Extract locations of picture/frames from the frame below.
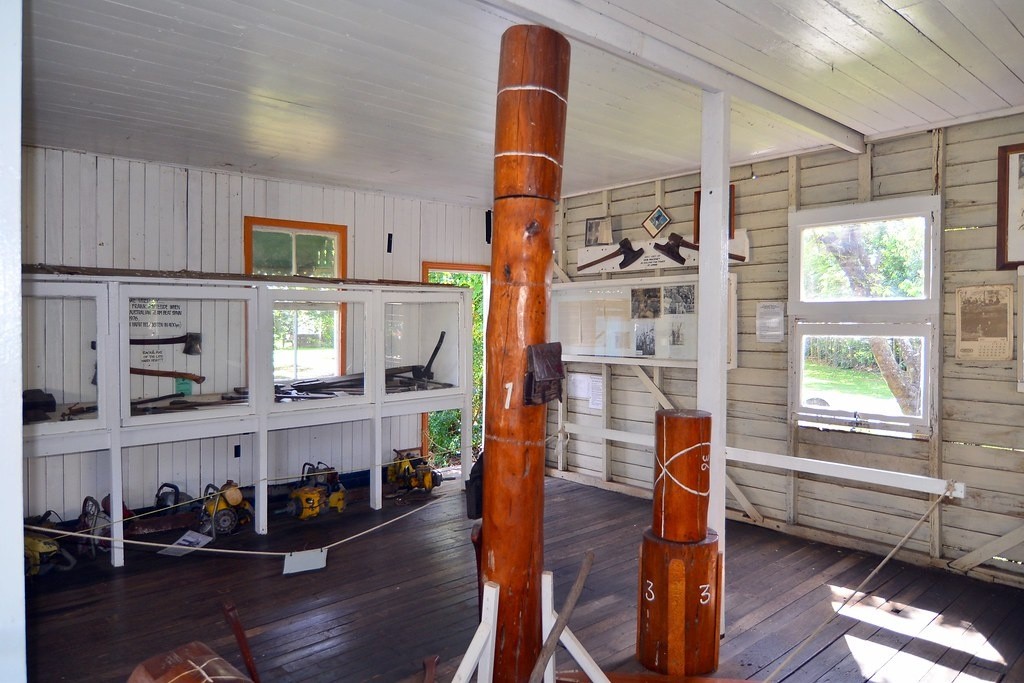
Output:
[641,205,673,239]
[996,143,1024,271]
[693,184,735,244]
[584,217,609,247]
